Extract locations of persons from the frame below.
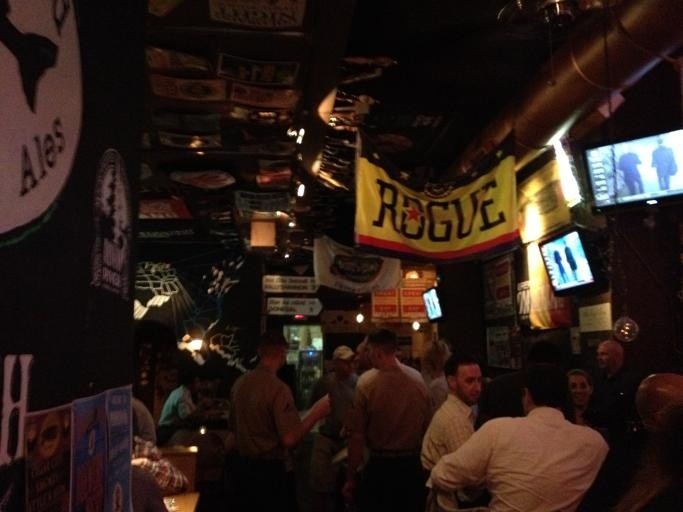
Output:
[563,240,577,281]
[130,397,156,456]
[419,354,482,511]
[639,404,681,510]
[420,341,450,408]
[564,368,593,423]
[427,359,610,511]
[326,331,434,511]
[192,399,224,492]
[227,329,332,510]
[618,153,644,196]
[308,346,372,512]
[651,138,677,190]
[157,371,196,444]
[128,437,192,511]
[587,340,635,455]
[554,251,569,285]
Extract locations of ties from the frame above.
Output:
[279,319,325,372]
[581,125,683,216]
[421,286,448,324]
[538,226,598,298]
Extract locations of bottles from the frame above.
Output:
[334,346,357,362]
